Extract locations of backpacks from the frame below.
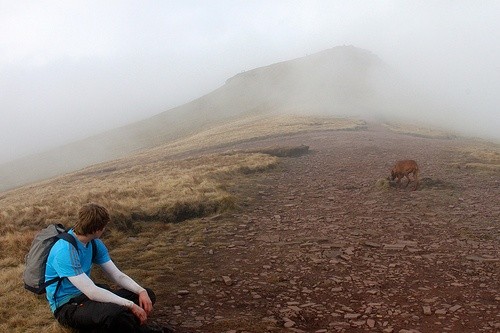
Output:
[23,222,98,295]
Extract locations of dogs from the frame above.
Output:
[390,159,420,188]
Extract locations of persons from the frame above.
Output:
[45,203,157,333]
